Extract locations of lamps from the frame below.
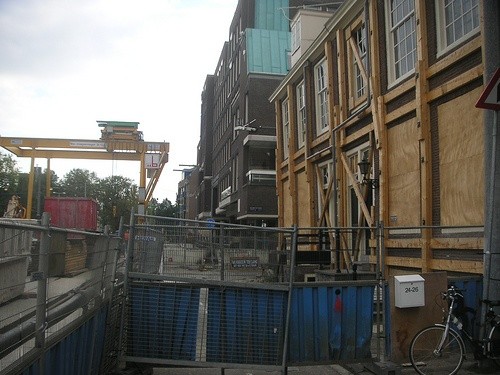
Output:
[358,159,370,174]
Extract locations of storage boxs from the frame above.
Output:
[44,197,98,230]
[395,275,424,308]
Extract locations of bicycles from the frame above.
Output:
[410,290,499,375]
